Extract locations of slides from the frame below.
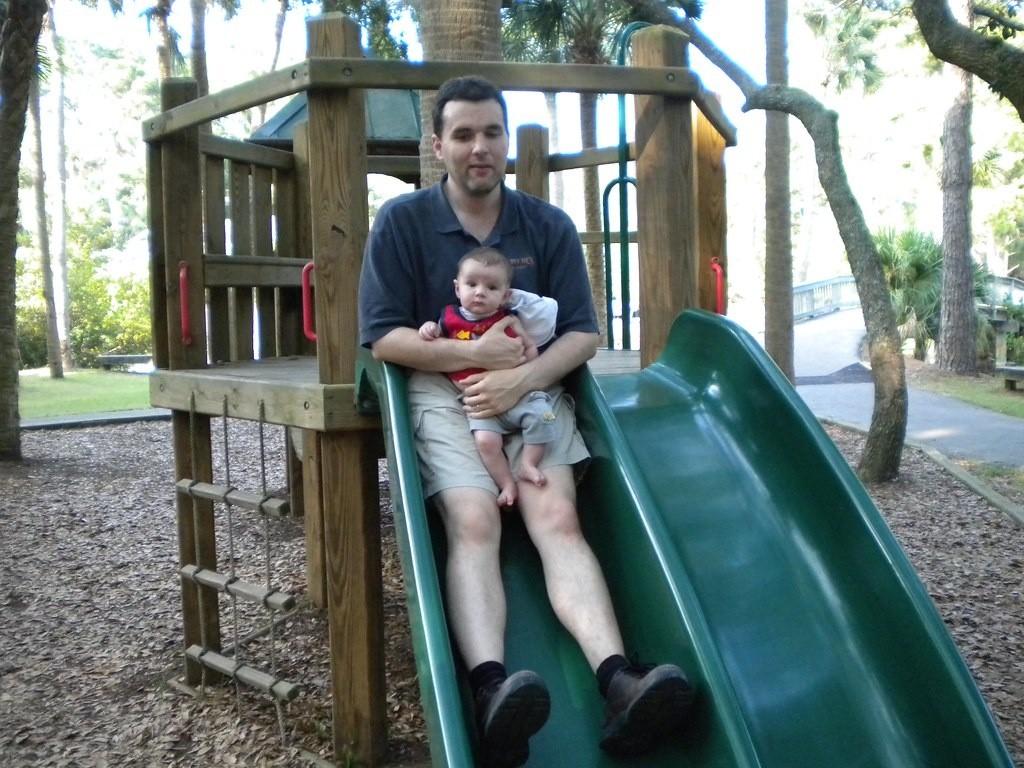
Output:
[352,307,1021,768]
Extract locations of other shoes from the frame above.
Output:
[599,664,692,767]
[473,671,551,767]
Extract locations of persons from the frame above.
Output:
[359,75,693,768]
[418,248,555,506]
